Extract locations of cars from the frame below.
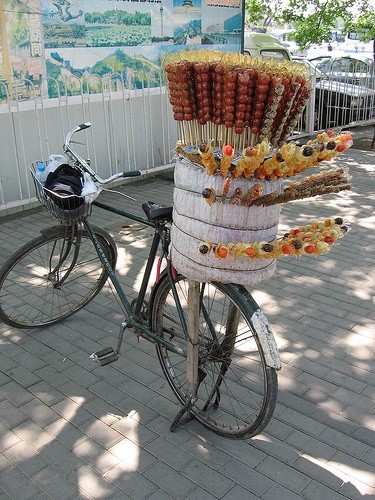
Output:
[244,24,375,131]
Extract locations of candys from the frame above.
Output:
[161,47,353,260]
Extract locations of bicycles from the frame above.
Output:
[0,120,283,441]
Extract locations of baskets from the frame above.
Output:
[27,153,92,226]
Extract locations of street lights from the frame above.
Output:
[160,4,164,37]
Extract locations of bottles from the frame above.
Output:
[35,163,49,190]
